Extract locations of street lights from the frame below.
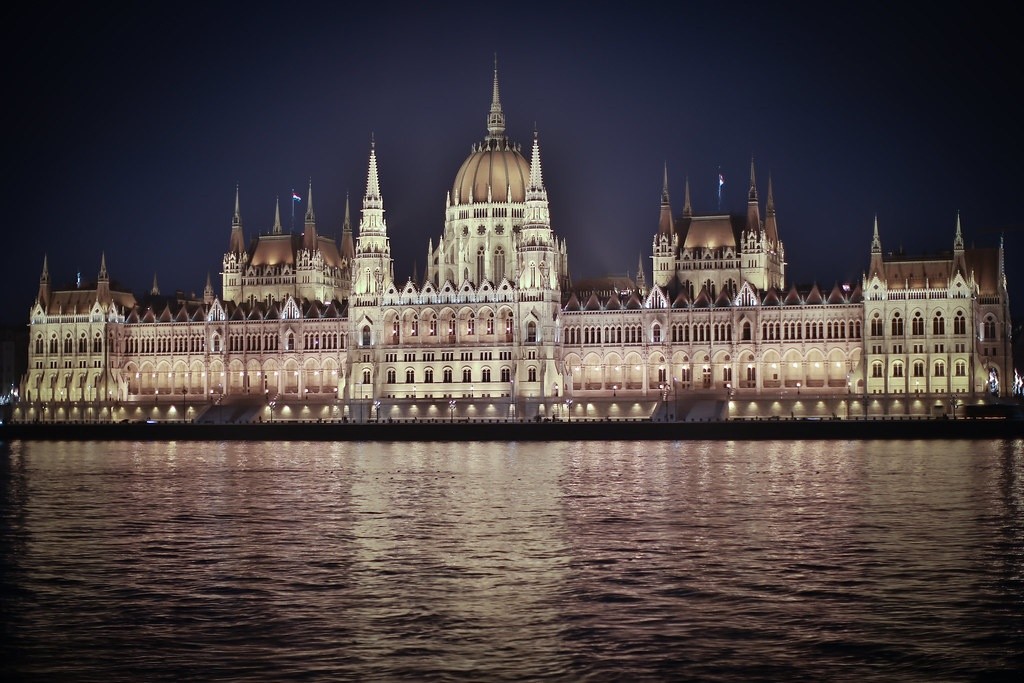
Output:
[89,386,91,425]
[449,400,455,423]
[373,400,381,422]
[567,399,573,421]
[673,377,676,421]
[40,402,47,425]
[862,394,870,420]
[269,401,276,424]
[510,381,514,422]
[950,395,959,419]
[359,381,363,423]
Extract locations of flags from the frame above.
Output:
[292,191,301,202]
